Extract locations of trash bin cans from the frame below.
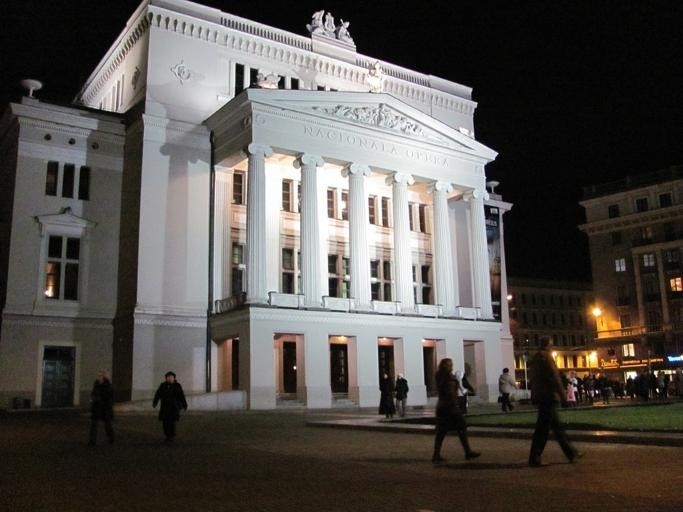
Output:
[13,397,30,410]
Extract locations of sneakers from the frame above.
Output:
[430,456,448,464]
[567,452,588,463]
[84,438,118,447]
[162,435,180,443]
[464,452,482,461]
[527,459,543,467]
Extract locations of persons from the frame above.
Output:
[563,367,682,403]
[497,367,518,411]
[377,373,395,419]
[391,372,409,417]
[523,334,586,466]
[82,366,116,448]
[151,371,188,440]
[430,358,480,464]
